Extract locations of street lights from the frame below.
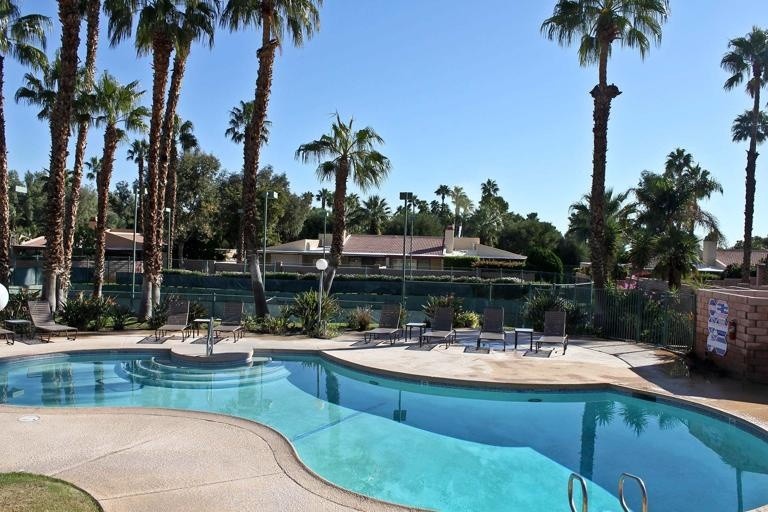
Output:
[315,258,328,325]
[164,208,171,269]
[132,184,148,299]
[399,191,413,311]
[262,190,278,292]
[323,211,330,259]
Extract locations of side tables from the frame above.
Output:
[192,318,210,338]
[4,319,31,345]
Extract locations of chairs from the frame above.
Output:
[0,328,15,345]
[154,300,191,343]
[212,302,244,342]
[27,297,77,343]
[363,304,568,357]
